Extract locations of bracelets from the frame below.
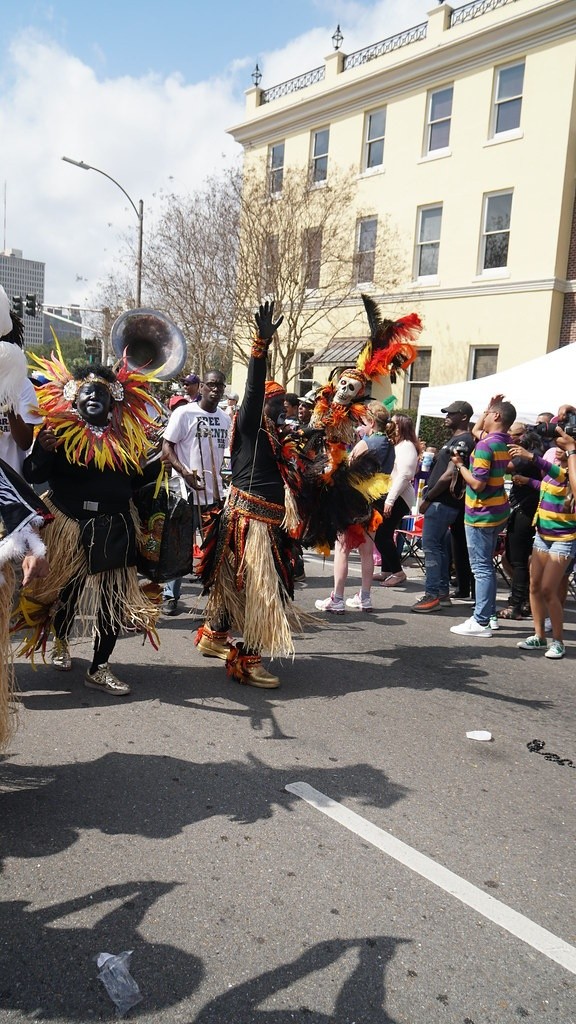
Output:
[424,496,432,502]
[457,464,466,472]
[251,337,274,358]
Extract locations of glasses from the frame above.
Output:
[483,409,500,418]
[203,381,227,391]
[181,382,193,388]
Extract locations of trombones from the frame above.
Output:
[192,419,221,543]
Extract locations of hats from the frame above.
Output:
[179,374,200,385]
[227,394,240,402]
[169,396,188,411]
[441,400,474,418]
[297,389,317,405]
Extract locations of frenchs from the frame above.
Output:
[110,308,189,382]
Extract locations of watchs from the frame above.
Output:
[565,449,576,457]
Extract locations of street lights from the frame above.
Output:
[62,156,143,308]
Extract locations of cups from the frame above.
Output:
[421,452,434,471]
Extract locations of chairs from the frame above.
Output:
[492,532,511,588]
[394,518,425,575]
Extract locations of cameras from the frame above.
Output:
[449,445,468,461]
[534,413,576,440]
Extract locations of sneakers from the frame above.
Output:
[314,590,346,615]
[347,592,371,613]
[51,633,71,671]
[83,665,131,695]
[410,588,565,657]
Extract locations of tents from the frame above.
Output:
[416,340,576,438]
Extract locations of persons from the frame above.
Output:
[315,400,396,615]
[410,401,476,614]
[496,403,576,658]
[0,285,60,752]
[194,300,330,688]
[22,325,168,697]
[282,389,319,580]
[373,414,420,587]
[418,438,438,463]
[161,370,234,616]
[225,393,241,415]
[167,374,203,412]
[449,394,516,638]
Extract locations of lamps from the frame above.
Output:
[332,24,344,50]
[251,64,262,86]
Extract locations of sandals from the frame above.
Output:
[380,572,406,588]
[372,571,388,581]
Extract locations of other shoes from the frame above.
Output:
[233,642,281,689]
[161,598,175,616]
[294,574,305,582]
[197,621,231,660]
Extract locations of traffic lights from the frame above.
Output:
[25,294,36,319]
[12,296,23,318]
[83,339,102,365]
